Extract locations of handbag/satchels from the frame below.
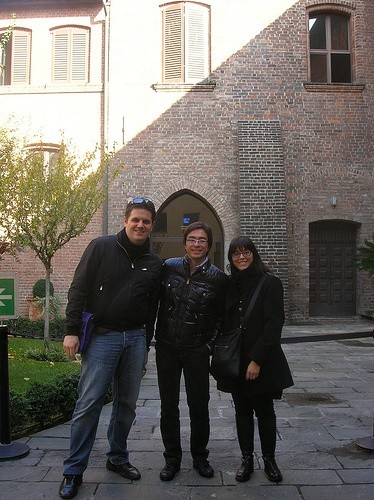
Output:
[78,311,96,353]
[210,330,246,382]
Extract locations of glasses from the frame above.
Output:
[131,198,154,209]
[186,238,208,244]
[232,249,252,257]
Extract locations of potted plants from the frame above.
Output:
[26,279,61,320]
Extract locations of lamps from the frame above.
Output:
[330,196,337,208]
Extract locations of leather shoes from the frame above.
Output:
[59,474,82,499]
[192,458,214,478]
[235,454,255,480]
[263,454,285,482]
[106,458,141,480]
[159,462,180,481]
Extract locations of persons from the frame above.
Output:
[217,235,296,482]
[57,194,163,500]
[152,221,230,482]
